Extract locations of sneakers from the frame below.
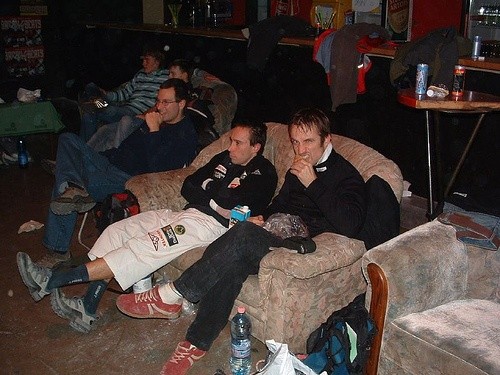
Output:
[17,251,52,303]
[116,283,183,318]
[159,338,206,375]
[41,250,72,271]
[48,187,96,215]
[50,288,100,334]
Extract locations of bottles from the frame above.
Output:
[191,6,197,28]
[426,83,449,98]
[229,306,252,375]
[18,141,29,169]
[480,3,500,22]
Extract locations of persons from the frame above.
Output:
[16,119,279,335]
[116,107,367,375]
[41,51,195,177]
[0,98,32,162]
[38,78,198,269]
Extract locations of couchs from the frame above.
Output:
[124,123,402,353]
[191,68,238,137]
[361,218,500,375]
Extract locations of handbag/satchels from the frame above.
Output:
[28,63,65,101]
[254,340,318,375]
[184,87,220,139]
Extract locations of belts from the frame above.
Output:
[437,212,500,251]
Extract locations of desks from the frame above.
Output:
[0,100,66,176]
[397,86,500,221]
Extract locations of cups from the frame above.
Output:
[208,13,217,32]
[133,274,154,295]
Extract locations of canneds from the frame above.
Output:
[414,63,429,95]
[451,65,466,96]
[472,35,481,58]
[415,94,426,101]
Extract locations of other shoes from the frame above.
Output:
[78,97,109,114]
[40,158,57,176]
[2,150,33,163]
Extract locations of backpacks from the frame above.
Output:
[94,189,140,234]
[302,290,381,375]
